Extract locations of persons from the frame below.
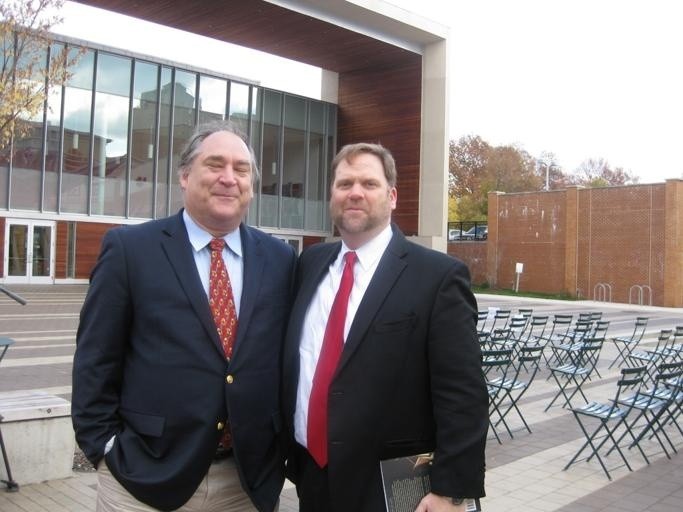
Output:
[277,141,486,512]
[70,123,300,511]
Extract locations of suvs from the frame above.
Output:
[454,225,487,241]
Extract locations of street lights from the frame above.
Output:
[536,157,559,191]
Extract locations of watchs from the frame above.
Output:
[441,493,469,506]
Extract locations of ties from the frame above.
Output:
[209,238,238,448]
[307,252,355,469]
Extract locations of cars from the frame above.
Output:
[449,229,465,240]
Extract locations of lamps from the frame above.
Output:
[148,102,154,159]
[72,134,79,149]
[272,126,276,175]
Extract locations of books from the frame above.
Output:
[379,451,481,512]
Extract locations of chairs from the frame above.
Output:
[0,286,26,492]
[478,308,683,481]
